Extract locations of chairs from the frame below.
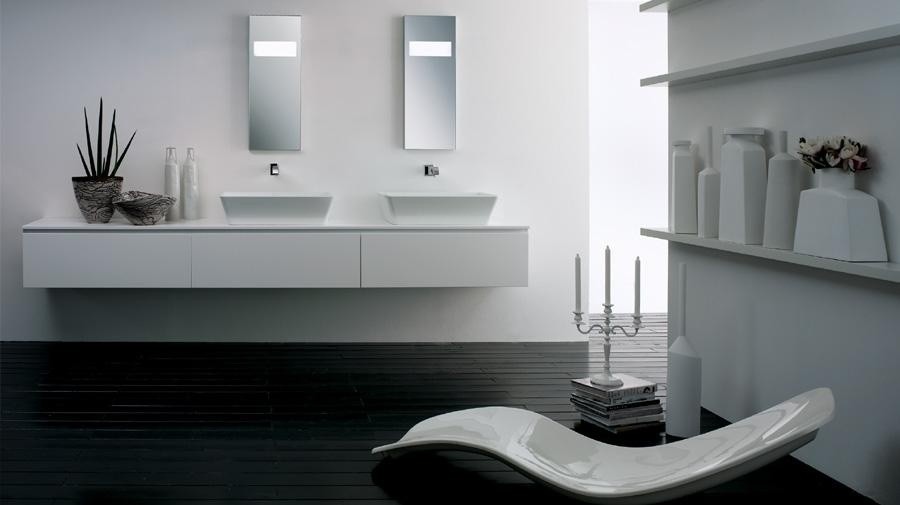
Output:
[371,386,835,499]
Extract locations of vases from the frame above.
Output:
[672,122,890,264]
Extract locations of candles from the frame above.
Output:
[604,245,612,305]
[575,253,581,310]
[634,256,641,314]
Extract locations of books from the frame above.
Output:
[570,373,665,436]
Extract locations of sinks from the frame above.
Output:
[218,194,335,227]
[376,191,498,228]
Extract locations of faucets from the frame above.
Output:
[424,165,439,176]
[269,163,279,175]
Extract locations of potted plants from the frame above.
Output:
[70,95,139,224]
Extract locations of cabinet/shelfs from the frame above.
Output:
[637,0,898,505]
